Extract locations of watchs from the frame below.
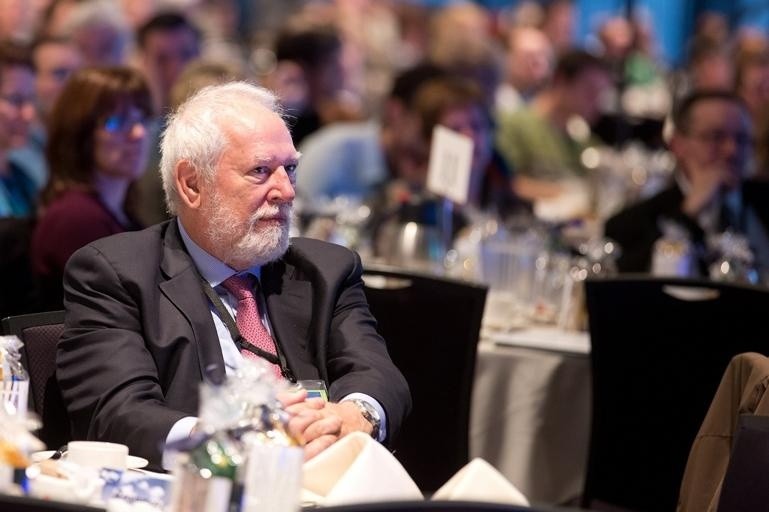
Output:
[355,398,381,444]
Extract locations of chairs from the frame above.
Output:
[574,274,769,509]
[359,266,494,485]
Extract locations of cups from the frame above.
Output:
[66,440,129,470]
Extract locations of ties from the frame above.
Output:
[716,202,738,236]
[219,274,289,384]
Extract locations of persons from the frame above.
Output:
[2,0,767,316]
[54,80,413,472]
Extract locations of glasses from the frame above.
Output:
[97,112,156,138]
